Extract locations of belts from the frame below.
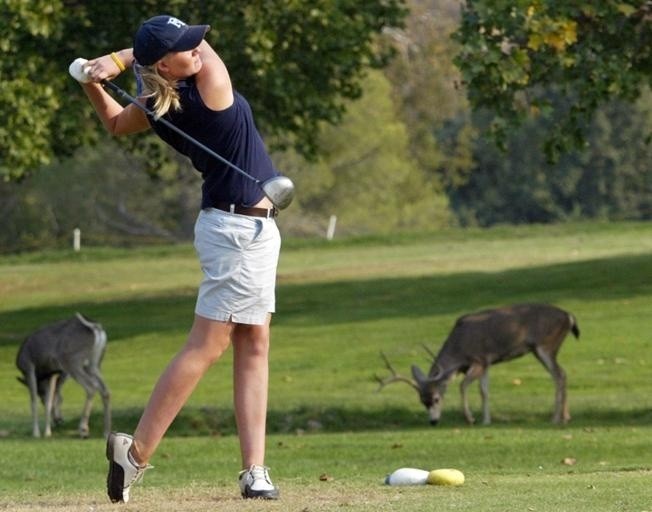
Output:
[218,204,279,219]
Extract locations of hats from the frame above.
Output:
[133,16,209,67]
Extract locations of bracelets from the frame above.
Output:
[109,50,126,73]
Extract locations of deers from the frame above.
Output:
[15,310,113,440]
[364,301,578,428]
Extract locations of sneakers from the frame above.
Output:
[238,466,278,500]
[107,431,145,503]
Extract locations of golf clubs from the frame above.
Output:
[82,64,294,210]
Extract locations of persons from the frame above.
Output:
[66,13,289,507]
